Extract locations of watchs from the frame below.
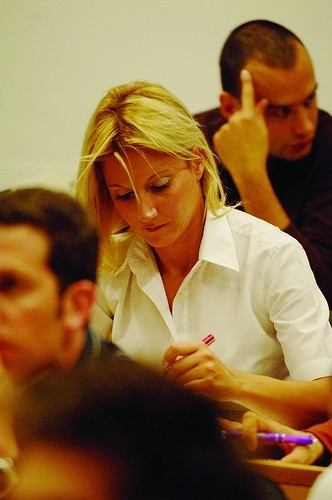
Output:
[0,450,30,498]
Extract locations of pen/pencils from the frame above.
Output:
[163,334,215,377]
[221,430,318,445]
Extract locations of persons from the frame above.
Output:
[0,183,156,500]
[216,409,332,465]
[68,79,332,430]
[0,365,290,500]
[190,19,331,308]
